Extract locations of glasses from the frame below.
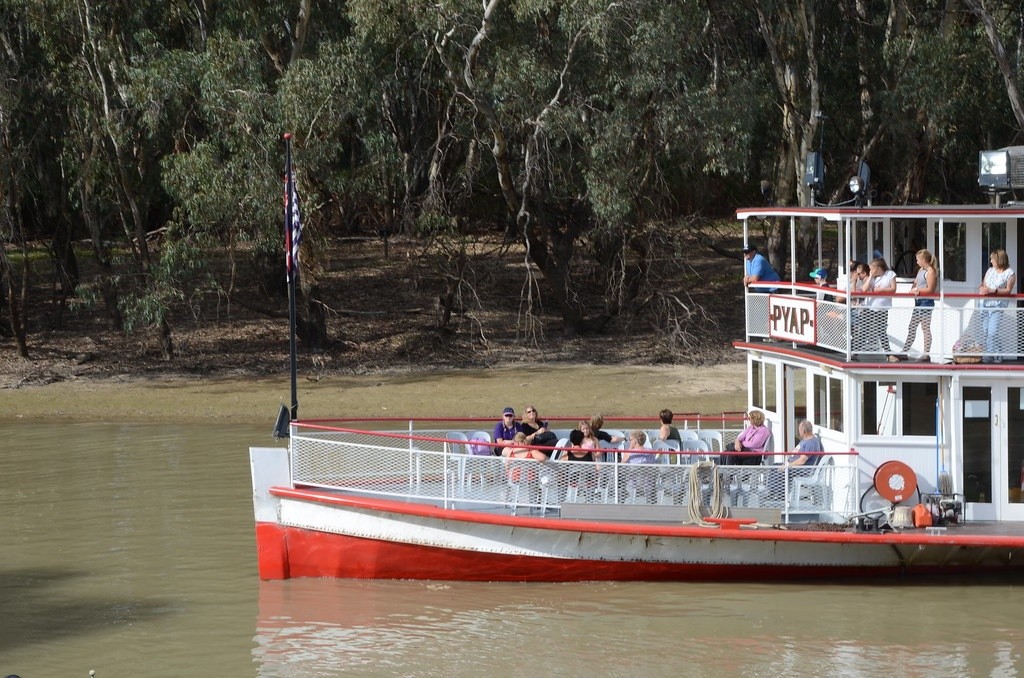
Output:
[856,271,864,276]
[526,409,536,414]
[993,249,1000,260]
[505,414,513,418]
[628,437,634,443]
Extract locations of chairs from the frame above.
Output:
[445,430,832,509]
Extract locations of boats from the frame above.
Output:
[244,132,1023,590]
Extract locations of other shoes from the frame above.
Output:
[982,355,991,364]
[763,338,768,342]
[993,356,1004,364]
[895,354,910,361]
[845,353,858,361]
[768,338,781,343]
[914,356,931,363]
[886,356,900,363]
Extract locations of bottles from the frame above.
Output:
[913,503,932,527]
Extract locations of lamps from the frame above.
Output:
[805,151,824,207]
[978,149,1017,206]
[855,160,873,205]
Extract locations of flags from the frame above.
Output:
[284,139,302,282]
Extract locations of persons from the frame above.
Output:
[716,411,770,484]
[892,248,938,363]
[835,257,899,362]
[621,431,655,490]
[657,409,683,464]
[767,421,820,500]
[979,249,1016,364]
[494,406,629,488]
[809,269,833,302]
[742,244,780,343]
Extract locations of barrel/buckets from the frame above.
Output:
[891,507,915,529]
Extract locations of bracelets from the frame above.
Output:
[914,288,919,296]
[995,289,998,294]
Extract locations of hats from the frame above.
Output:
[503,408,515,416]
[809,269,828,280]
[741,243,756,255]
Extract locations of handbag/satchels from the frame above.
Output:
[534,431,557,446]
[953,335,982,364]
